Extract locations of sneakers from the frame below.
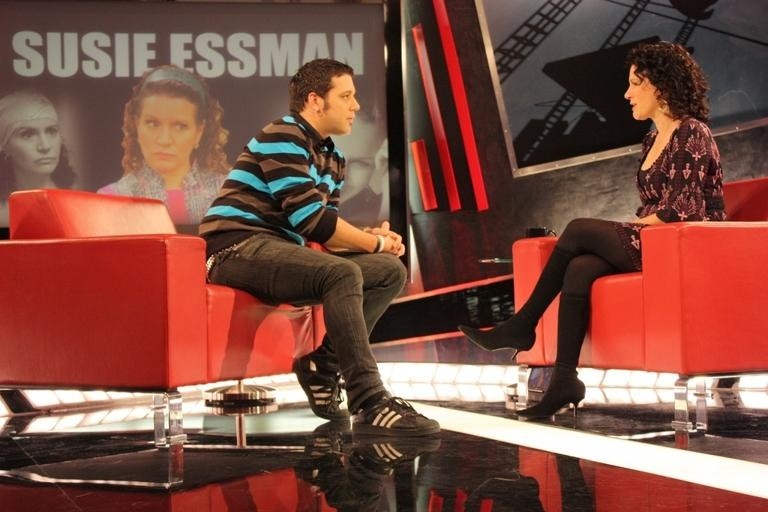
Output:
[352,434,442,477]
[294,354,350,419]
[293,420,351,484]
[349,392,440,437]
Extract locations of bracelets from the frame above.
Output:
[373,237,381,255]
[377,235,386,254]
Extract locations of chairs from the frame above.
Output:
[505,223,715,434]
[0,188,329,450]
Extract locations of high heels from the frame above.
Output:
[459,317,536,360]
[515,365,585,418]
[467,468,539,501]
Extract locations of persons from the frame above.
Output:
[298,431,440,512]
[196,55,444,436]
[329,103,391,228]
[0,86,78,230]
[97,63,236,224]
[552,452,595,512]
[457,38,729,419]
